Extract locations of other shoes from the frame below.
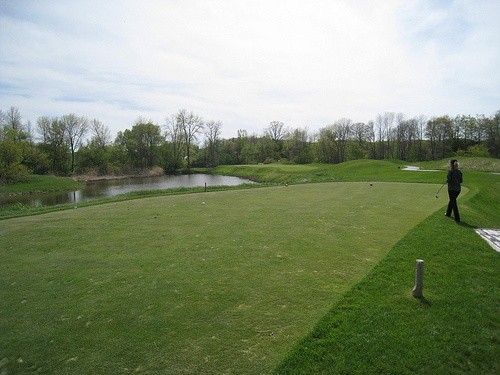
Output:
[453,218,460,223]
[444,212,450,217]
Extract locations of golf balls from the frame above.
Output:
[201,201,205,205]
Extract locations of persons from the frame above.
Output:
[444,159,464,223]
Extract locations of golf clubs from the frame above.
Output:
[436,180,447,198]
